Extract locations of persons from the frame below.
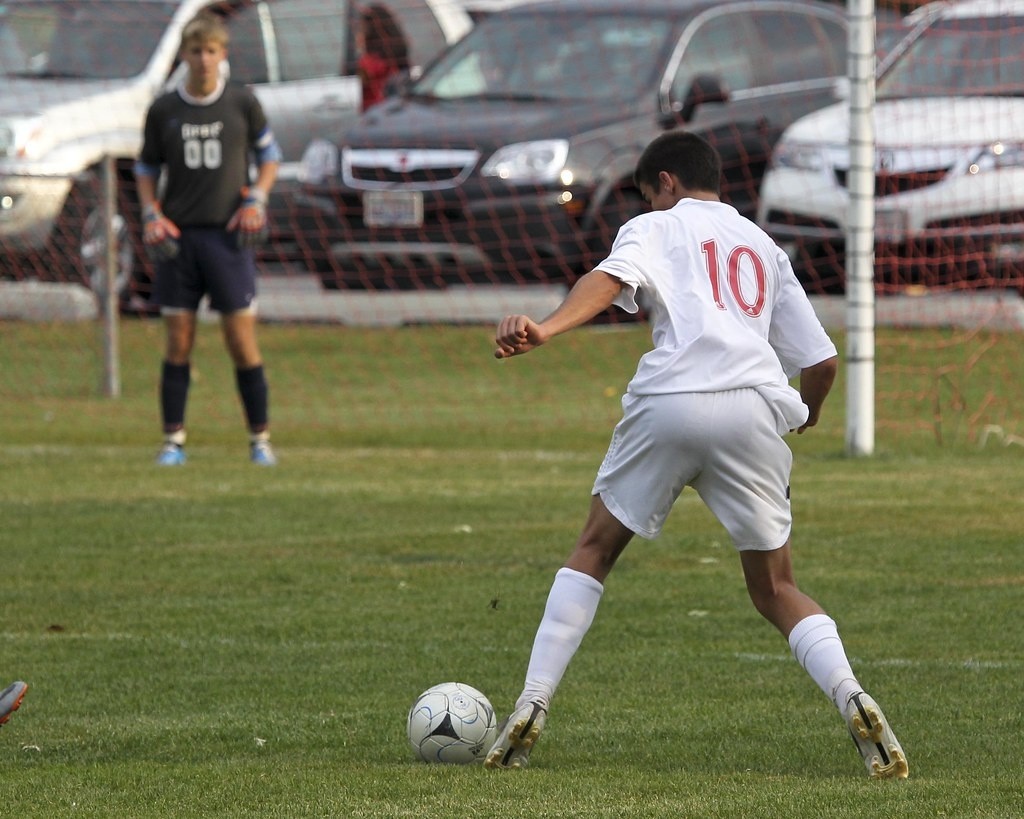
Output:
[135,14,283,467]
[353,0,414,114]
[1,681,27,726]
[482,132,910,782]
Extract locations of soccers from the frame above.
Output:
[406,681,497,764]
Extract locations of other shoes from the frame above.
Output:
[249,440,278,466]
[154,441,186,467]
[0,681,29,729]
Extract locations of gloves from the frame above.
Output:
[226,186,270,252]
[141,199,181,262]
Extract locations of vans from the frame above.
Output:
[0,0,475,290]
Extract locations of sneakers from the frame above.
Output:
[844,690,909,780]
[482,694,549,770]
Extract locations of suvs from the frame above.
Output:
[760,0,1024,300]
[296,0,897,288]
